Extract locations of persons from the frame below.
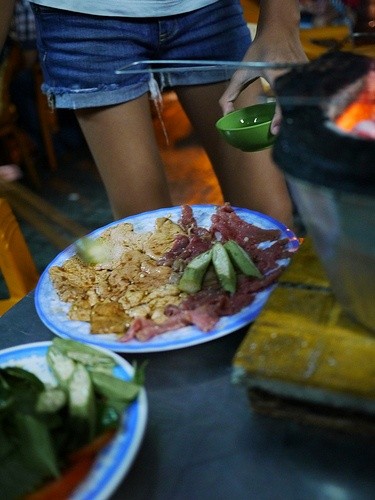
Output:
[30,0,309,236]
[0,0,37,54]
[297,0,375,37]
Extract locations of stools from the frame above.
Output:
[0,159,39,319]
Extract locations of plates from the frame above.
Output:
[34,202,300,354]
[0,341,149,500]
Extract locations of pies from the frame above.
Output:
[48,217,192,334]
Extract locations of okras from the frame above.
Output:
[0,338,148,500]
[180,241,264,292]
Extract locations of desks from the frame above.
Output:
[0,237,375,500]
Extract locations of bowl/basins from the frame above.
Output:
[215,103,278,152]
